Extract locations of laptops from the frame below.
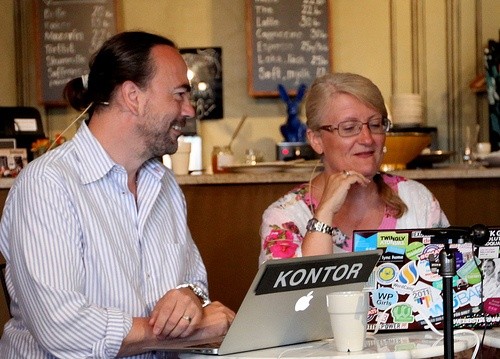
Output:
[351,226,500,333]
[155,249,384,355]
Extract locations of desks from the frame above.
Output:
[177,328,500,359]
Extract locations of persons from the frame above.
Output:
[0,31,236,359]
[258,72,450,270]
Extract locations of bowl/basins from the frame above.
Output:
[380,125,438,172]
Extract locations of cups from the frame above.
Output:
[326,291,369,352]
[169,142,191,176]
[390,93,423,128]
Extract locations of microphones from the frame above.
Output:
[423,223,490,245]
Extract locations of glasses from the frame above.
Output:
[315,117,392,139]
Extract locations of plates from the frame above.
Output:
[419,153,450,163]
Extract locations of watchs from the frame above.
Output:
[306,218,333,236]
[176,283,211,307]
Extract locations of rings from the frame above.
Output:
[182,315,191,323]
[343,170,349,176]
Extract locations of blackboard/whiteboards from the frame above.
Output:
[245,0,333,97]
[32,0,125,106]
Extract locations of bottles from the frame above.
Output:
[211,145,233,173]
